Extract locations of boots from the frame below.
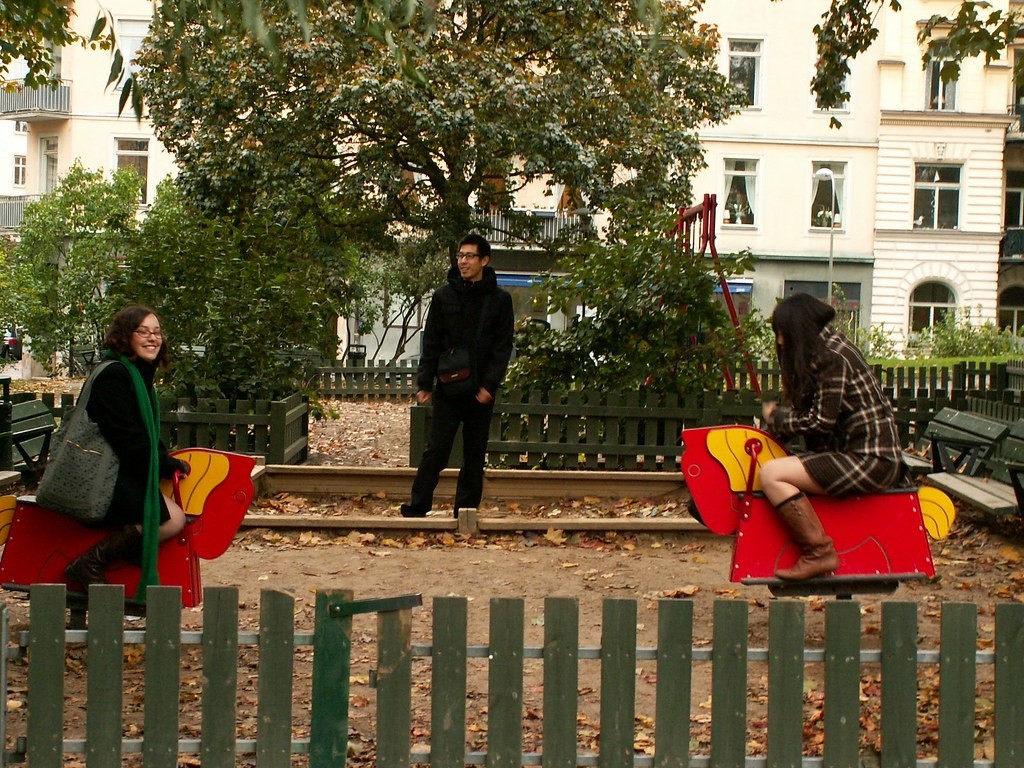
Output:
[775,491,838,581]
[65,523,142,599]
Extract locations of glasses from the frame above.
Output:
[455,252,479,259]
[132,328,164,340]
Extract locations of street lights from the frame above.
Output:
[816,168,835,306]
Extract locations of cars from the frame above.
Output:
[0,325,22,360]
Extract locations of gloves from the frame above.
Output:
[159,455,188,481]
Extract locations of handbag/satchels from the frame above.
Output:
[434,346,470,383]
[35,405,119,522]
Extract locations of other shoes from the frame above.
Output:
[400,504,426,517]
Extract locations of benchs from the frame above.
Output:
[11,394,59,478]
[901,408,1024,532]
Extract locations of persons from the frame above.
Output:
[401,234,515,519]
[759,293,903,580]
[69,306,191,597]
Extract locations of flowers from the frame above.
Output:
[817,211,832,219]
[732,203,749,213]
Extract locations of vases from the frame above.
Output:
[735,213,744,223]
[822,218,828,227]
